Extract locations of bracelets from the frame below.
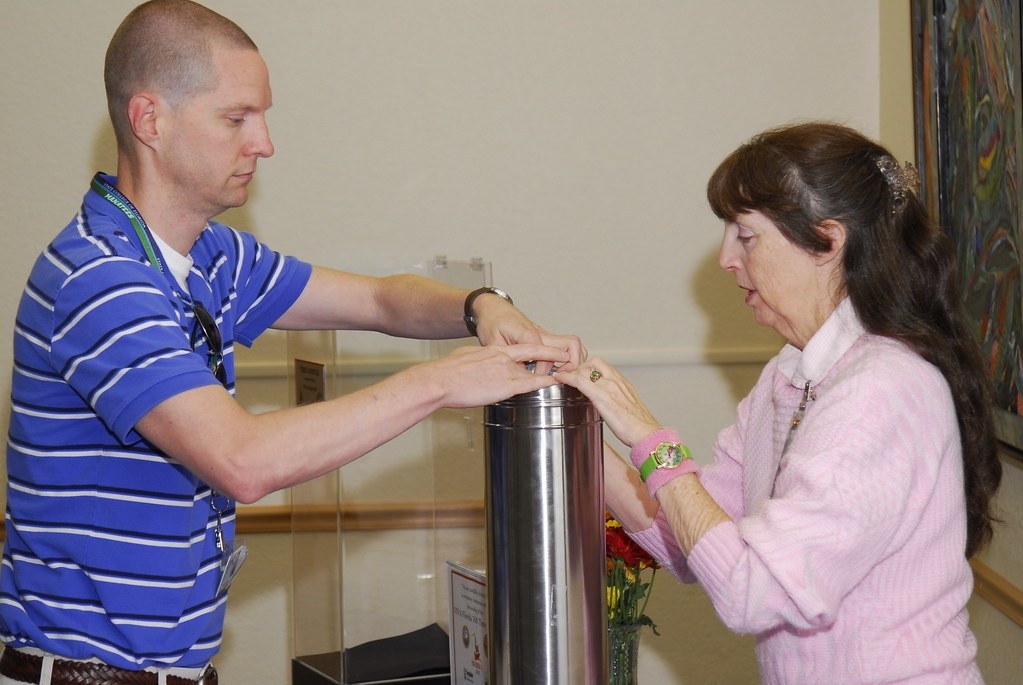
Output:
[629,427,702,502]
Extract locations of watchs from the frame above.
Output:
[462,286,514,338]
[638,441,695,484]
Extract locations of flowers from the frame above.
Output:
[604,508,662,638]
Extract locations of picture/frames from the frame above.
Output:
[910,1,1023,455]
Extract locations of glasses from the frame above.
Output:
[191,304,228,388]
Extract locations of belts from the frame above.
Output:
[0,645,218,685]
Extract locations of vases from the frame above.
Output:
[608,624,642,685]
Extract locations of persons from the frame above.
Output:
[551,122,1005,685]
[0,0,589,685]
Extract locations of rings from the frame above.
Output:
[588,369,603,383]
